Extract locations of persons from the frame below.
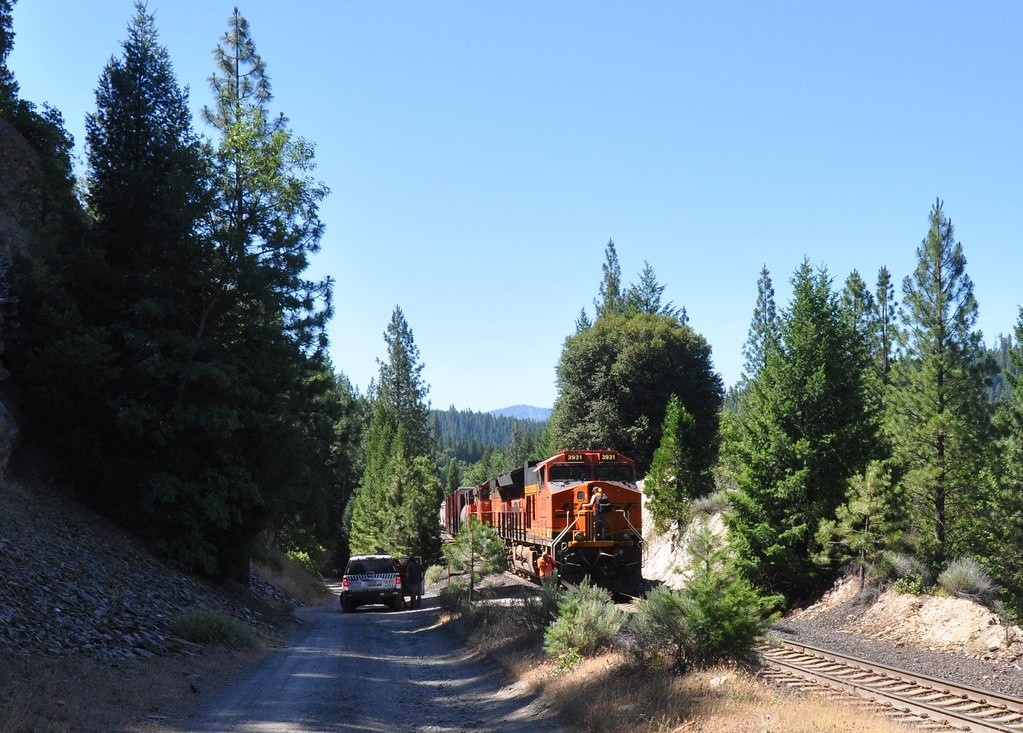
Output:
[406,555,428,609]
[582,486,607,541]
[537,552,558,588]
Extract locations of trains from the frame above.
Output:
[439,449,647,590]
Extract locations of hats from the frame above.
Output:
[592,487,598,492]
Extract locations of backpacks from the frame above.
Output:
[596,493,611,513]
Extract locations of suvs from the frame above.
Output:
[340,555,424,612]
